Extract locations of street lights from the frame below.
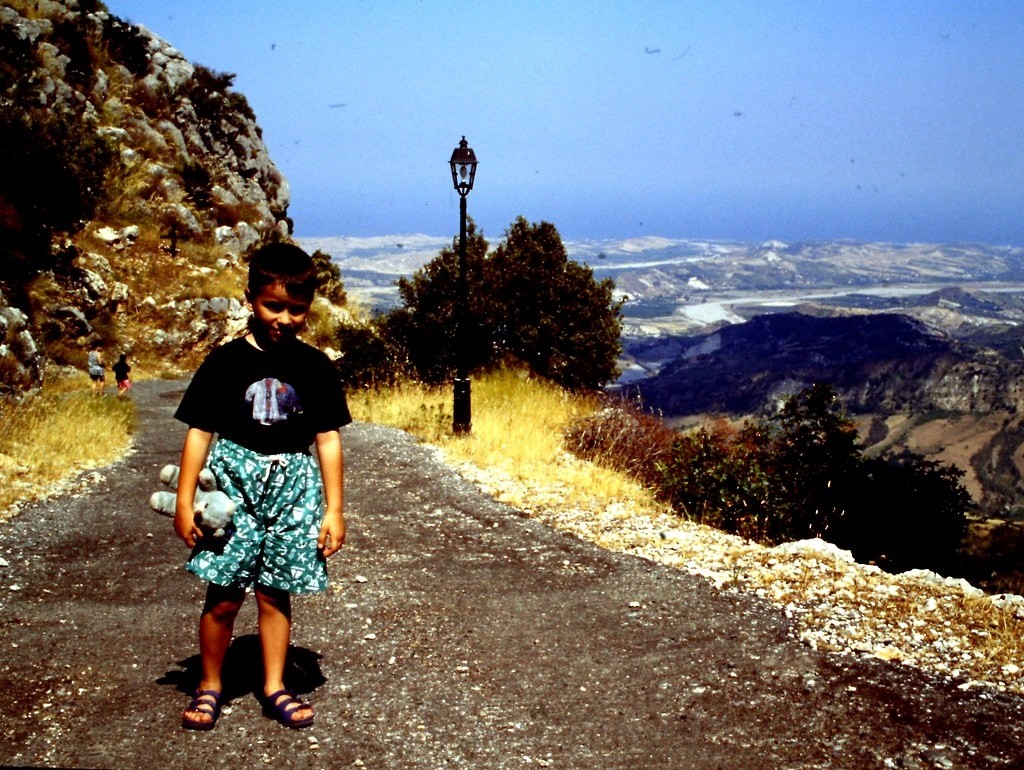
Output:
[449,137,480,434]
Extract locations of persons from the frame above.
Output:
[88,340,107,401]
[106,354,131,400]
[174,243,353,730]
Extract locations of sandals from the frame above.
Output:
[183,690,221,731]
[263,690,314,727]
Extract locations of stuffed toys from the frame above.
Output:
[150,464,236,535]
